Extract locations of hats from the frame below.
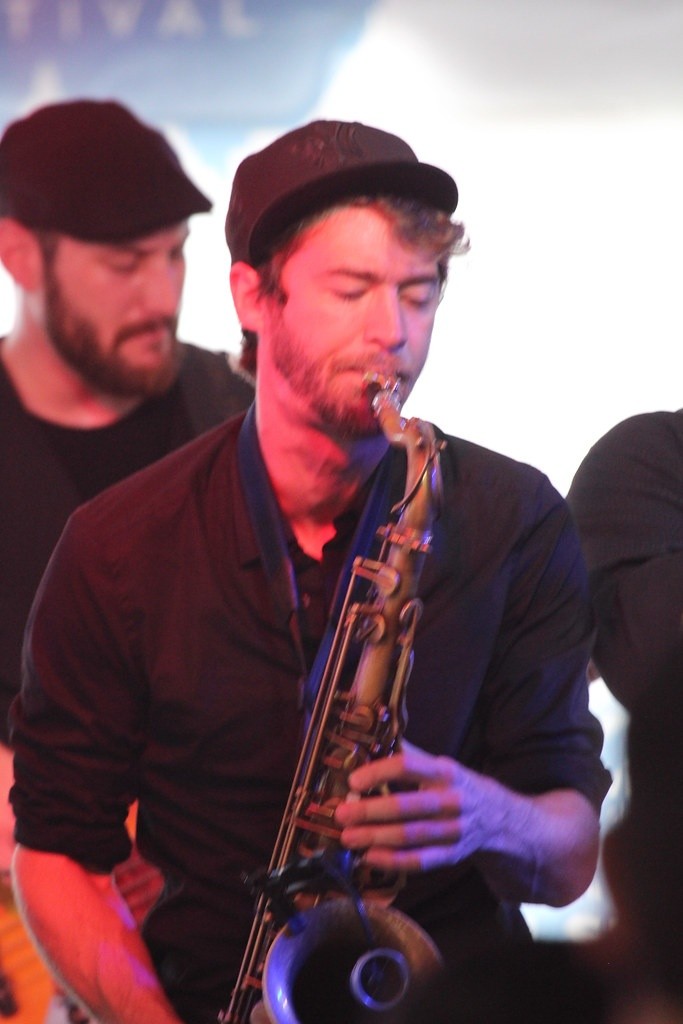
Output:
[223,121,459,268]
[0,101,214,247]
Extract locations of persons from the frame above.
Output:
[7,116,611,1024]
[0,98,256,1024]
[563,407,683,988]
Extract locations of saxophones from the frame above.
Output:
[217,379,446,1024]
